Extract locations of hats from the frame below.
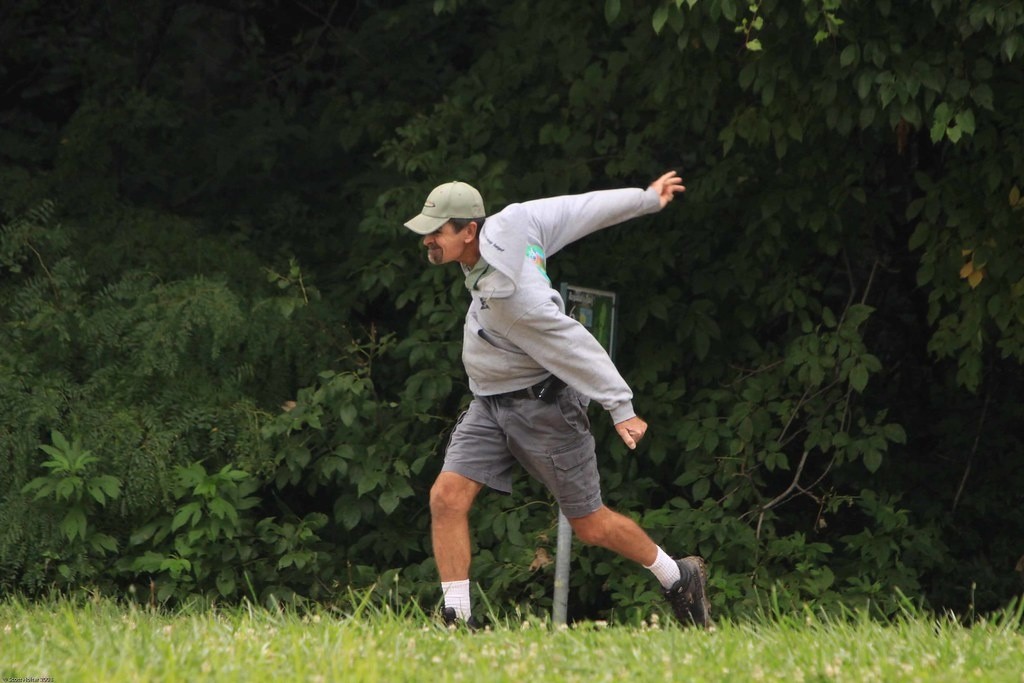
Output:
[403,181,486,234]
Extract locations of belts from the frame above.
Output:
[503,386,541,400]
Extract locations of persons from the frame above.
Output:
[402,170,708,635]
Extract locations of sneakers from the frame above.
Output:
[659,556,711,632]
[440,607,481,630]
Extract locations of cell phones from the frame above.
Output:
[537,375,560,401]
